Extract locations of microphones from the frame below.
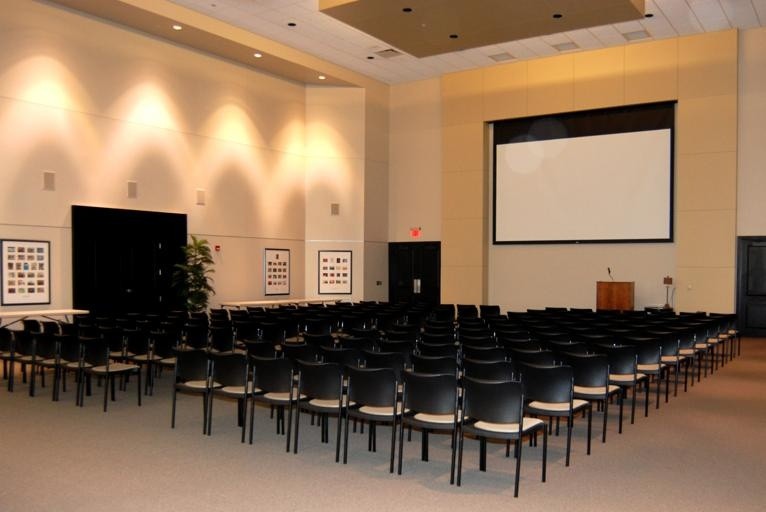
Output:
[607,267,616,282]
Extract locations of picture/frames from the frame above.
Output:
[0,239,51,306]
[264,248,290,295]
[318,250,352,294]
